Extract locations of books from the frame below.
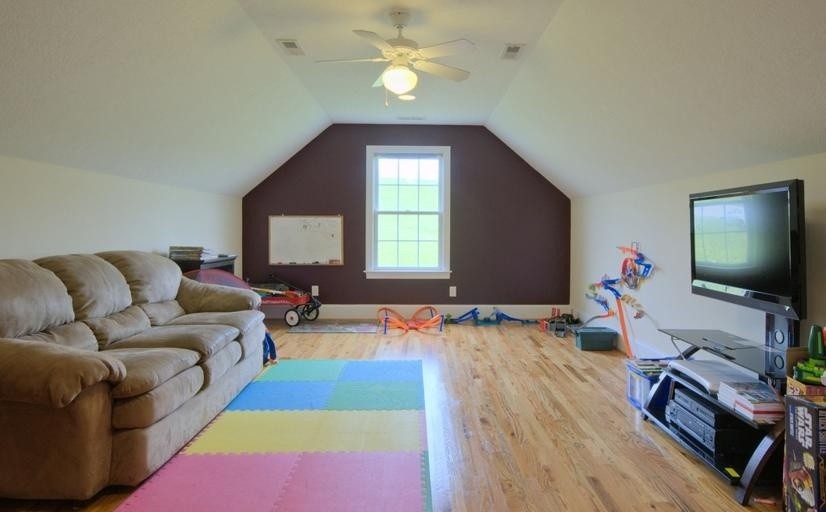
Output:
[716,380,787,423]
[168,247,217,261]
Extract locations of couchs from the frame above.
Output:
[1,252,266,502]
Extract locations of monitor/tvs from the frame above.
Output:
[689,178,807,321]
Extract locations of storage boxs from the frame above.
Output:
[576,326,618,352]
[626,355,694,410]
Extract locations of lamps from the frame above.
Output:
[382,67,416,95]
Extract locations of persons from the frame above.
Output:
[263,323,278,367]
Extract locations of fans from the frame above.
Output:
[316,24,476,88]
